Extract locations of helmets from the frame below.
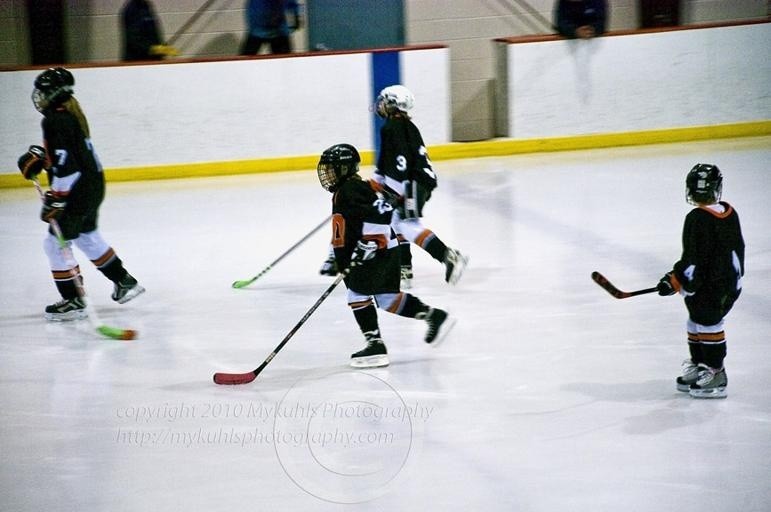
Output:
[317,144,360,192]
[374,86,415,119]
[32,67,75,117]
[685,163,724,206]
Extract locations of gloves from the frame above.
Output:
[656,269,682,297]
[351,240,382,264]
[320,259,339,277]
[18,145,47,180]
[41,190,66,222]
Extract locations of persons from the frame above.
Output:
[237,1,306,56]
[373,85,459,282]
[17,65,137,315]
[117,1,180,63]
[655,161,746,390]
[553,1,609,39]
[317,144,448,360]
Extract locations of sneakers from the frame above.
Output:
[400,266,414,279]
[424,306,449,345]
[689,364,728,389]
[443,247,462,284]
[45,296,87,314]
[350,340,390,359]
[676,359,699,384]
[111,273,138,301]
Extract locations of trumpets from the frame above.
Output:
[590,271,660,299]
